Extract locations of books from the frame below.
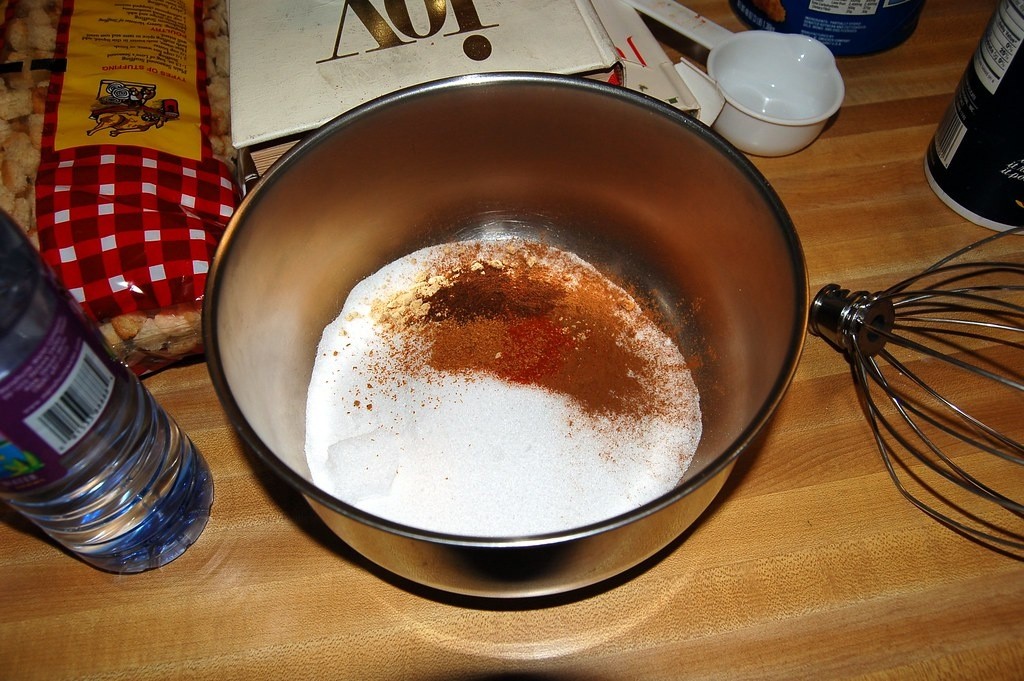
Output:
[228,1,701,177]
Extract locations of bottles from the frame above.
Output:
[0,203,215,575]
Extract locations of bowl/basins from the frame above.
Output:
[708,30,845,156]
[201,73,809,599]
